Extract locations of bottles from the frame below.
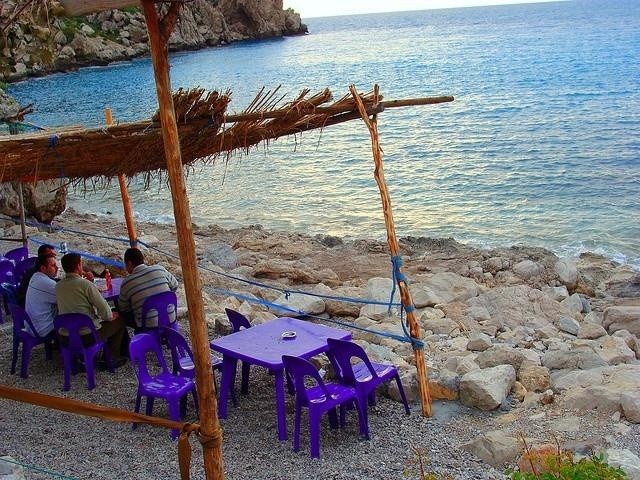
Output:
[105,269,113,289]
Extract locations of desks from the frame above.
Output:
[93,277,126,302]
[209,316,352,440]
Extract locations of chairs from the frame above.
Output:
[326,337,410,430]
[128,333,198,438]
[282,354,370,459]
[9,304,54,378]
[0,246,38,315]
[162,325,239,417]
[140,292,187,357]
[225,308,250,331]
[53,312,114,391]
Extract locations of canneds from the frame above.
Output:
[60,242,67,251]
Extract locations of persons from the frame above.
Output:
[17,244,127,368]
[111,247,179,357]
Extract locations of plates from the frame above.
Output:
[283,332,296,338]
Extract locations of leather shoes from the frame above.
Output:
[101,359,126,368]
